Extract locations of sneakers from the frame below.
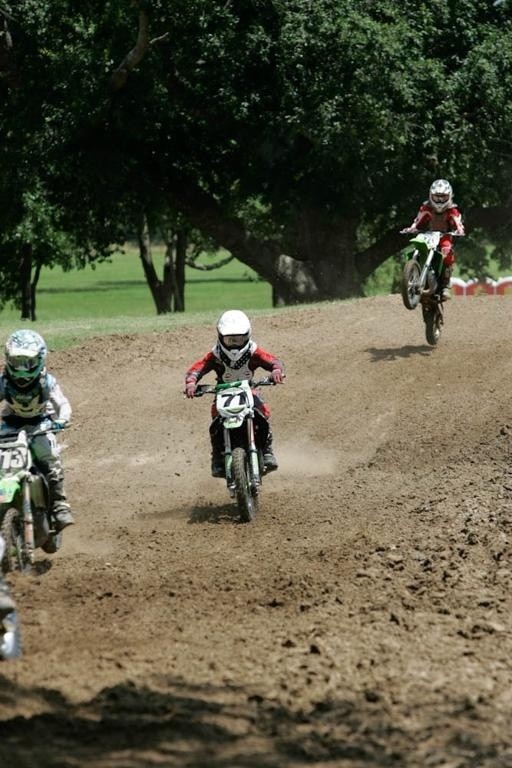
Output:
[263,453,278,474]
[54,507,76,527]
[212,462,225,478]
[442,288,451,299]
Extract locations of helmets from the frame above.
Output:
[429,179,452,212]
[4,329,47,389]
[216,309,251,363]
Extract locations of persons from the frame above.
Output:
[402,179,466,301]
[0,330,73,527]
[0,536,22,661]
[181,309,284,477]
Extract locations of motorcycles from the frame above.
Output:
[0,421,72,576]
[183,372,287,522]
[399,227,466,345]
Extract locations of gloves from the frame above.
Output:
[185,375,197,399]
[51,419,66,435]
[269,365,283,385]
